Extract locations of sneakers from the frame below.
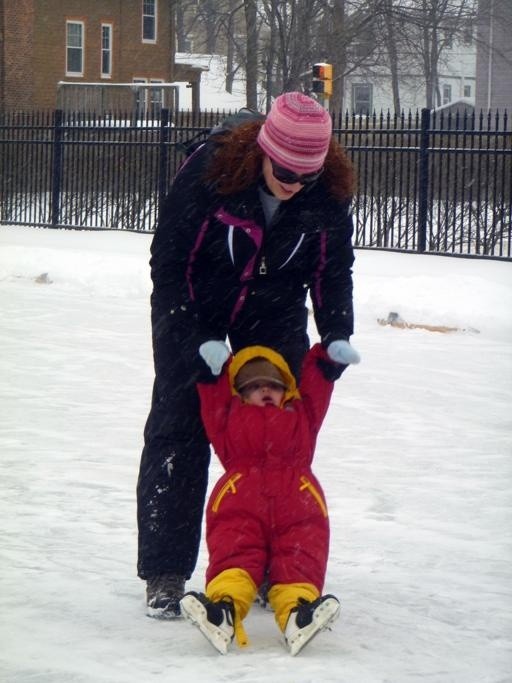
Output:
[180,594,237,654]
[285,593,340,656]
[145,574,187,619]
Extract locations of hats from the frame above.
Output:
[258,91,333,172]
[234,356,290,392]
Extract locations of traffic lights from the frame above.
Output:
[312,62,333,99]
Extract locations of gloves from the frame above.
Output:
[198,340,231,377]
[327,339,361,365]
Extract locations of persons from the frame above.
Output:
[177,339,361,657]
[137,92,357,620]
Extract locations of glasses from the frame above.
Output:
[268,154,325,186]
